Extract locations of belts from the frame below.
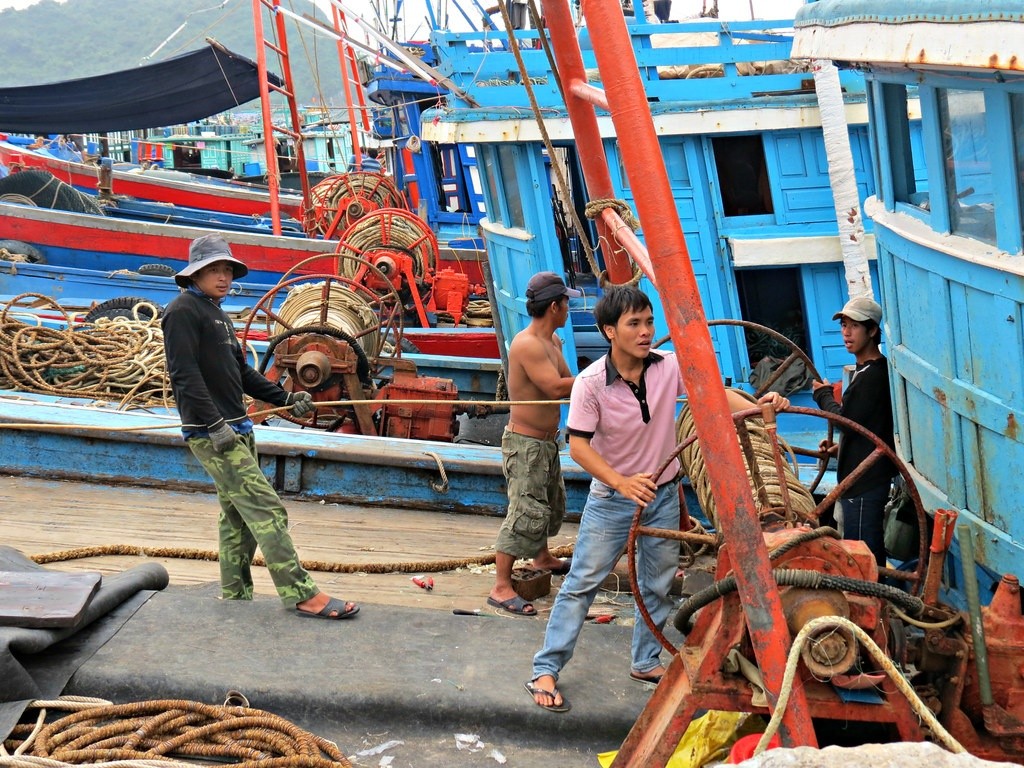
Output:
[507,421,560,443]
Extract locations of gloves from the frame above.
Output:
[285,390,318,419]
[209,422,237,455]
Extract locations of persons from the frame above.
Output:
[486,273,582,615]
[161,233,360,619]
[348,147,384,174]
[525,287,790,711]
[811,295,901,676]
[183,148,201,164]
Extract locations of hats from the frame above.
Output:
[832,297,882,326]
[175,232,248,289]
[527,271,582,302]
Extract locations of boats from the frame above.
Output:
[2,13,1023,617]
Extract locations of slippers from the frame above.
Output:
[629,671,663,686]
[549,560,571,575]
[487,594,537,616]
[295,596,361,620]
[522,680,572,712]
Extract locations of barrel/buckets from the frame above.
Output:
[151,160,163,169]
[304,158,319,171]
[244,162,262,176]
